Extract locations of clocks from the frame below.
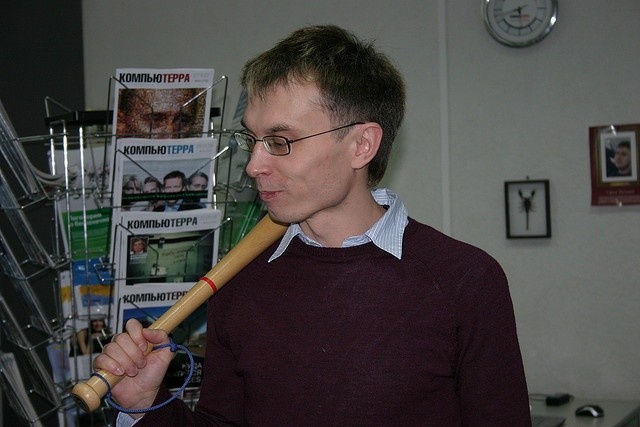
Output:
[482,0,558,48]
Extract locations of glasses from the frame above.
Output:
[234,121,365,155]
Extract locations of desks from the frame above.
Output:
[529,394,640,427]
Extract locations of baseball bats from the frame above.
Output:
[72,214,290,412]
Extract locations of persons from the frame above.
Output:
[160,169,187,212]
[131,236,147,254]
[605,138,631,176]
[186,171,209,209]
[72,317,106,355]
[122,173,144,211]
[140,175,165,211]
[119,90,199,139]
[92,23,530,422]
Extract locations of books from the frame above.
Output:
[46,140,110,427]
[108,65,215,193]
[108,135,219,272]
[110,205,226,330]
[112,282,211,414]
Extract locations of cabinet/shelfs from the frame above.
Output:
[0,73,256,427]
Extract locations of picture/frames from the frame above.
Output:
[589,124,640,206]
[504,179,552,239]
